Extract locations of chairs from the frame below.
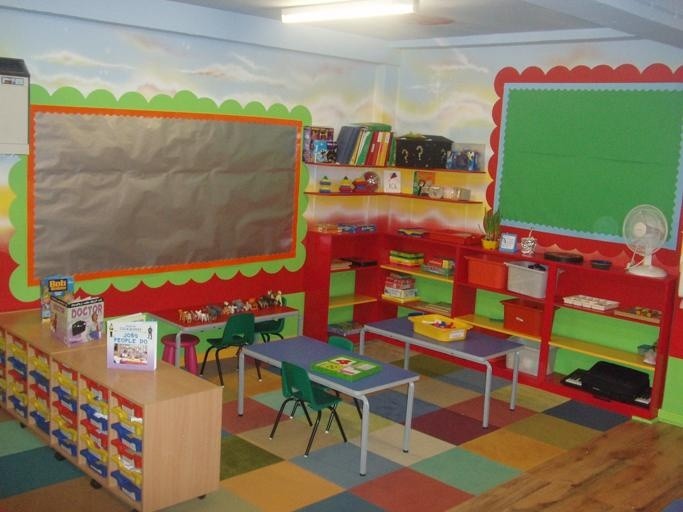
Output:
[269,362,347,457]
[312,336,367,419]
[201,313,262,388]
[252,296,287,344]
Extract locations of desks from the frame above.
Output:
[360,316,525,430]
[237,336,420,476]
[142,298,302,368]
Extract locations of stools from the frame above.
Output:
[161,333,199,375]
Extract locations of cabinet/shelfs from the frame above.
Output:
[0,308,222,512]
[381,225,680,419]
[303,217,400,344]
[303,125,486,207]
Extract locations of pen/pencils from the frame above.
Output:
[528,228,533,238]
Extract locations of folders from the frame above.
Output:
[334,123,396,166]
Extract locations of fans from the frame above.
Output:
[622,204,669,279]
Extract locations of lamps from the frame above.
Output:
[281,0,419,26]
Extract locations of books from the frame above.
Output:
[303,126,336,165]
[413,171,436,196]
[51,295,104,344]
[382,273,418,304]
[39,276,73,320]
[384,171,400,193]
[419,255,453,275]
[389,249,424,270]
[106,313,157,371]
[312,221,375,235]
[563,294,620,311]
[335,123,394,166]
[331,260,352,271]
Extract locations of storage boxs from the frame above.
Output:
[505,260,560,297]
[407,314,474,341]
[499,298,560,337]
[505,334,558,375]
[465,252,514,289]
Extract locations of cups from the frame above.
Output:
[520,237,537,259]
[444,185,454,199]
[426,184,443,200]
[448,186,462,200]
